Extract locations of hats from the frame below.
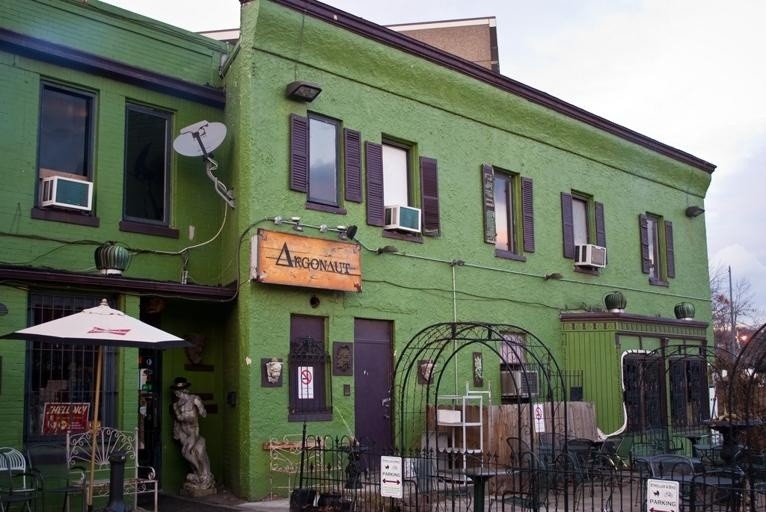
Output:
[170,376,192,391]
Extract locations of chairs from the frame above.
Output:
[505,431,766,512]
[411,458,470,512]
[0,447,45,512]
[269,432,343,502]
[65,425,159,512]
[26,443,87,512]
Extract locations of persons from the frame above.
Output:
[170,377,210,483]
[0,299,184,512]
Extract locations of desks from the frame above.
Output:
[435,468,520,512]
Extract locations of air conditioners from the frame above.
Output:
[384,205,422,234]
[41,176,93,213]
[574,244,606,268]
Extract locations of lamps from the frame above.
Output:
[546,273,563,280]
[686,206,705,218]
[501,370,539,396]
[347,225,358,240]
[378,246,398,253]
[451,260,465,267]
[286,80,322,103]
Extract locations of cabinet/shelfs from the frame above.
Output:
[431,393,484,487]
